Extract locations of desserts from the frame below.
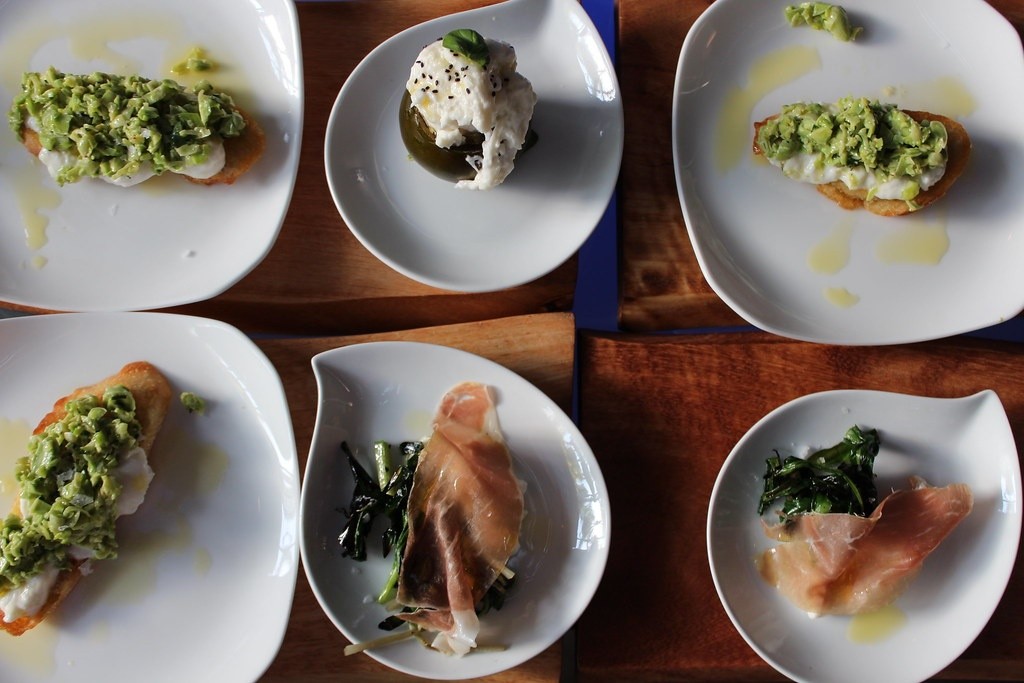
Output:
[399,29,538,188]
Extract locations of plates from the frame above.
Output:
[0,0,306,312]
[300,340,613,681]
[671,0,1024,347]
[0,312,300,683]
[323,0,625,293]
[706,388,1024,683]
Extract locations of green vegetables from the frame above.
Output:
[757,426,881,531]
[337,436,519,656]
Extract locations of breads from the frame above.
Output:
[22,104,266,185]
[754,108,972,216]
[0,362,171,638]
